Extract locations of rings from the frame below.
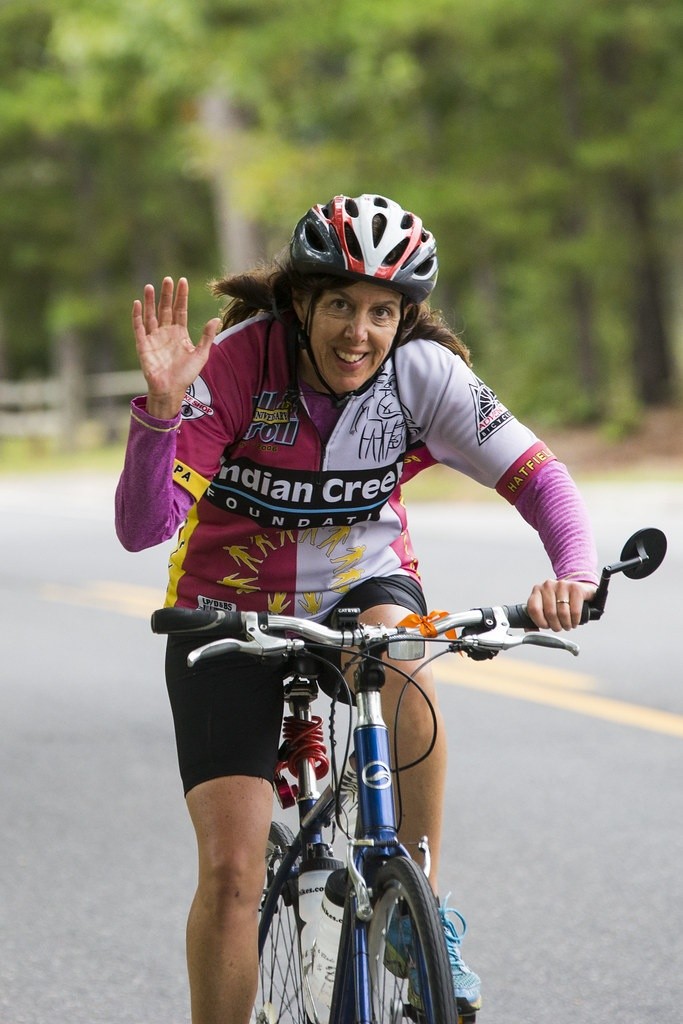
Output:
[557,600,569,603]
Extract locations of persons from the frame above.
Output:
[115,193,600,1024]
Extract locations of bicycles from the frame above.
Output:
[151,527,666,1024]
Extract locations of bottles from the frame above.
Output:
[298,842,352,1024]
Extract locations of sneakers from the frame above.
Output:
[384,902,482,1015]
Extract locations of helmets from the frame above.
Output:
[288,192,439,304]
